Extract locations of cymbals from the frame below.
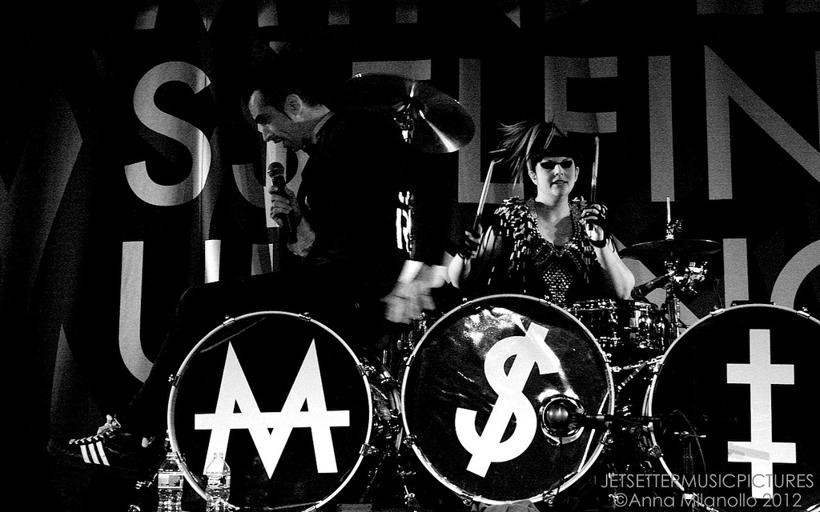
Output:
[617,238,719,257]
[344,72,474,153]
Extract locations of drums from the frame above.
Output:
[399,295,647,506]
[570,296,670,363]
[641,304,819,511]
[167,313,400,512]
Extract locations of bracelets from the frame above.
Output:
[589,238,610,248]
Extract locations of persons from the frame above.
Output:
[46,77,407,470]
[447,120,635,302]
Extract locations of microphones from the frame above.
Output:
[263,161,299,244]
[631,272,670,301]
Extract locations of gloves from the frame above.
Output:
[580,203,610,248]
[458,225,482,258]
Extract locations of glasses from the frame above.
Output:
[540,159,574,169]
